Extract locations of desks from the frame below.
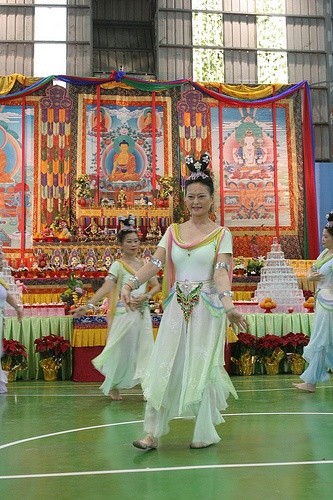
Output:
[3,314,238,382]
[233,312,330,375]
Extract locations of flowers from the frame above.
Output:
[59,274,86,302]
[235,332,310,362]
[247,255,267,272]
[1,338,29,373]
[34,334,71,359]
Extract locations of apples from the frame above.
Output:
[38,253,48,267]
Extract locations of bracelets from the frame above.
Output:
[88,302,93,309]
[147,292,152,298]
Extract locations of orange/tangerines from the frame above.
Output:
[303,296,315,308]
[260,297,276,309]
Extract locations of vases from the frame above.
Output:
[235,349,305,375]
[64,302,74,316]
[40,356,62,381]
[2,361,18,382]
[247,265,264,276]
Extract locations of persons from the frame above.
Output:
[0,278,23,393]
[118,190,127,203]
[121,154,250,449]
[68,213,162,402]
[293,213,333,392]
